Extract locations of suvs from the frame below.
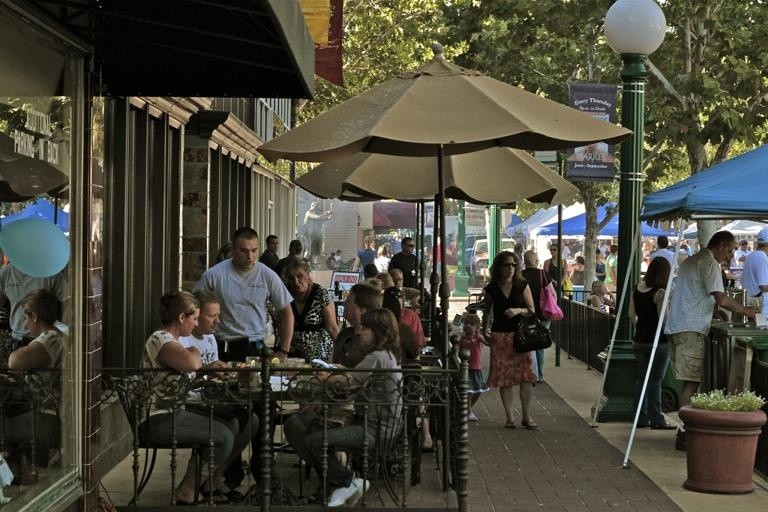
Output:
[468,238,525,285]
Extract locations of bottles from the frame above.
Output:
[341,262,361,272]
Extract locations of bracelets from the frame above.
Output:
[482,340,486,344]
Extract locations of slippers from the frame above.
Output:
[506,422,516,428]
[522,421,538,427]
[172,481,244,507]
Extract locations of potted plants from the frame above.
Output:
[678,388,768,495]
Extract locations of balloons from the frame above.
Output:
[0,218,70,278]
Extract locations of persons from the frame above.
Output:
[283,284,383,505]
[663,230,758,451]
[299,201,336,258]
[175,285,259,503]
[191,227,294,490]
[481,251,538,428]
[457,314,490,422]
[1,287,70,487]
[381,287,433,450]
[627,256,677,430]
[0,262,69,351]
[304,308,403,507]
[215,227,767,364]
[135,290,230,507]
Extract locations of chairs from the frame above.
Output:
[335,391,409,506]
[403,358,423,486]
[6,366,62,485]
[115,373,202,505]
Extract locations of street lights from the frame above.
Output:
[600,0,664,427]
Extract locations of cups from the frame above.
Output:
[228,356,305,382]
[342,291,347,301]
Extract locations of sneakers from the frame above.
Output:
[676,426,686,450]
[309,477,370,507]
[469,412,478,421]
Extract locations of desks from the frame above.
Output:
[333,295,347,325]
[207,359,344,498]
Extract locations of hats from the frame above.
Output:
[757,229,768,244]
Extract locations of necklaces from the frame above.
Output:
[164,327,176,338]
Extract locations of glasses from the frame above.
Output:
[550,249,557,251]
[406,243,415,248]
[502,263,517,267]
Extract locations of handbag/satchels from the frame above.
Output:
[563,279,574,296]
[517,307,552,353]
[540,287,564,320]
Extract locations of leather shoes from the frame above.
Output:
[652,423,677,429]
[636,423,650,427]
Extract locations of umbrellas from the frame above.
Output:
[255,44,633,491]
[294,147,581,337]
